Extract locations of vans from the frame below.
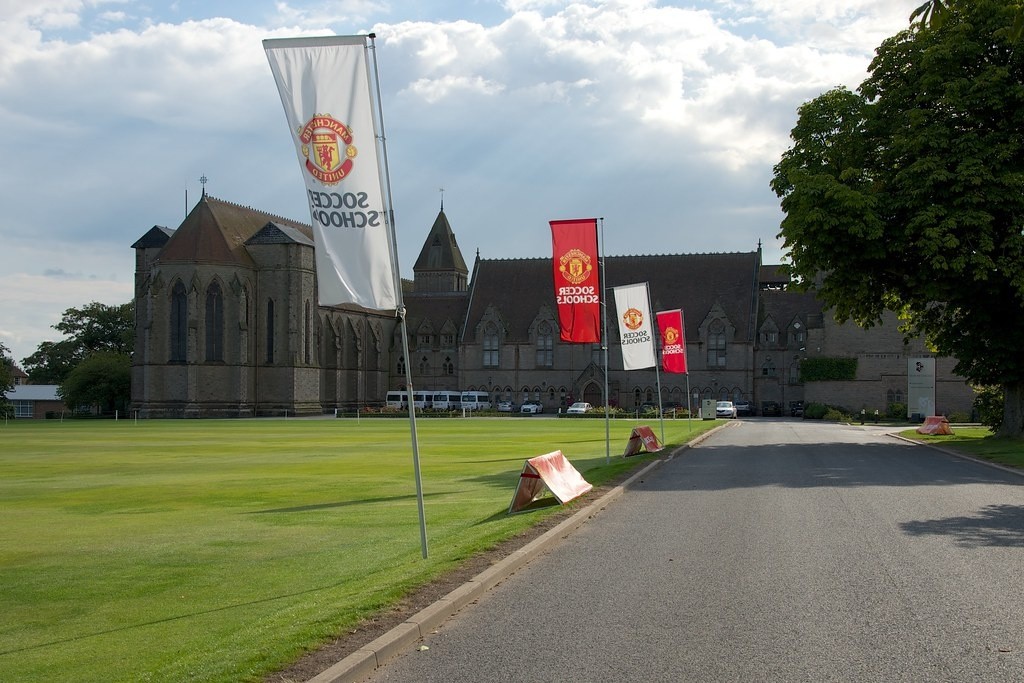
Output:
[386,391,409,410]
[413,390,433,410]
[461,391,493,411]
[431,391,461,411]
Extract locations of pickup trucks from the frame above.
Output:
[716,401,737,419]
[735,401,751,416]
[762,400,782,417]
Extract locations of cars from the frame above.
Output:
[520,400,543,414]
[567,402,593,413]
[791,401,803,416]
[639,402,660,418]
[663,402,687,410]
[497,400,518,412]
